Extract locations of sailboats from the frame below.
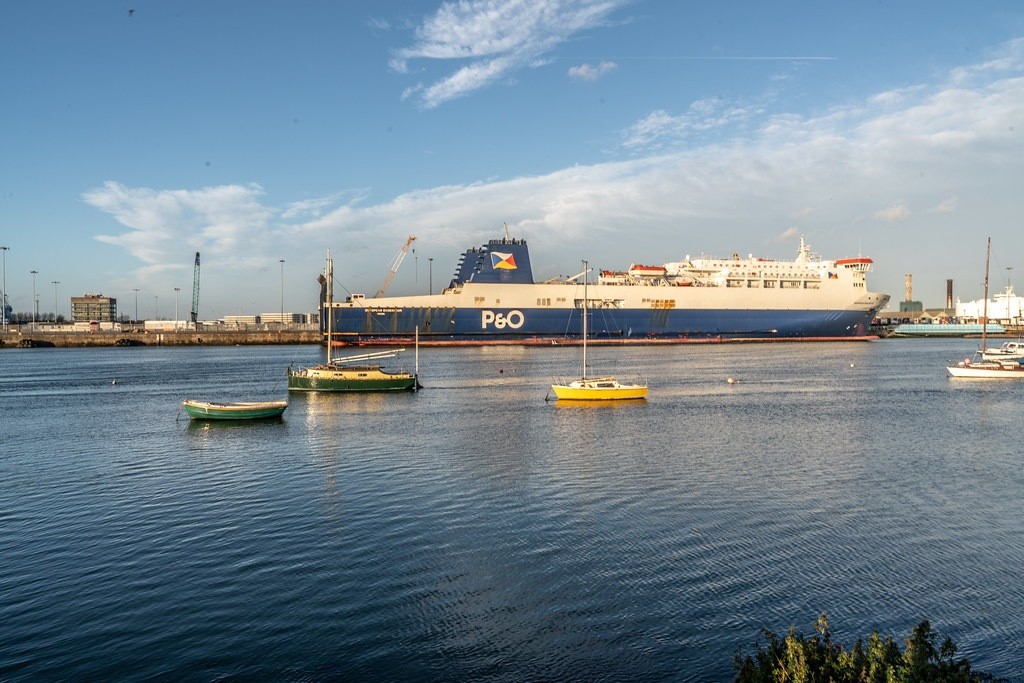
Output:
[945,233,1024,379]
[546,258,652,400]
[286,247,423,392]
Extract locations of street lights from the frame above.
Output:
[134,288,140,324]
[280,259,288,331]
[175,288,181,331]
[52,281,59,324]
[31,270,39,330]
[1,246,10,331]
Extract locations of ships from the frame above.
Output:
[316,223,891,348]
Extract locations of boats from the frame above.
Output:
[183,399,289,421]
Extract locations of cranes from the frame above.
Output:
[191,251,201,323]
[373,235,419,300]
